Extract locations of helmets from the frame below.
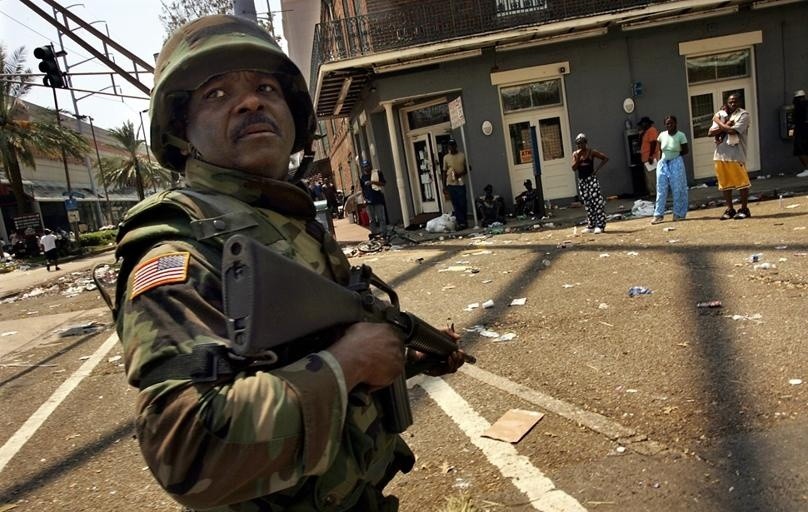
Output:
[150,13,318,175]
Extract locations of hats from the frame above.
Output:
[636,117,654,124]
[361,159,370,166]
[447,139,456,145]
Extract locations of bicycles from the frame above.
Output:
[356,221,406,253]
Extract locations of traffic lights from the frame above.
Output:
[32,42,64,90]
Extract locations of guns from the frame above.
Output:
[222,234,476,434]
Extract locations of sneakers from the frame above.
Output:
[651,216,664,224]
[720,208,750,219]
[581,227,604,234]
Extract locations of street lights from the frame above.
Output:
[138,108,156,193]
[80,114,114,225]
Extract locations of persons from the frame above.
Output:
[793,90,808,178]
[359,160,390,241]
[651,115,689,223]
[93,14,464,512]
[636,117,660,200]
[708,93,751,219]
[512,178,538,215]
[476,184,504,225]
[712,104,729,144]
[572,133,609,234]
[442,138,471,229]
[307,177,338,219]
[0,226,74,271]
